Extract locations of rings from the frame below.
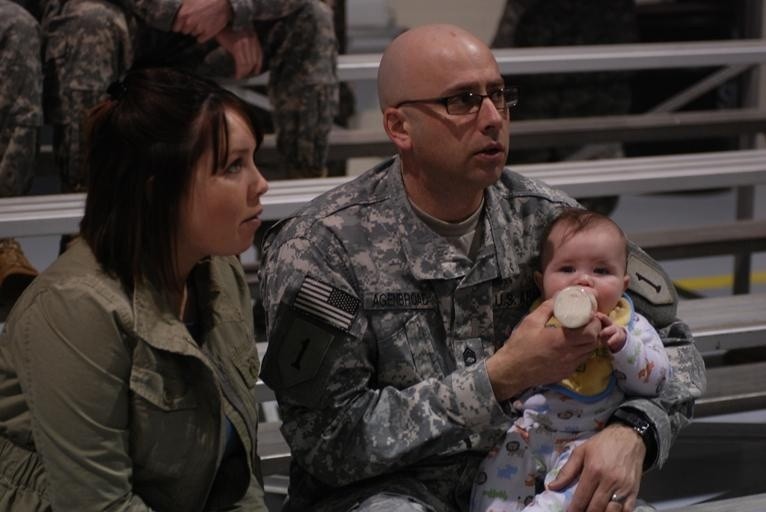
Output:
[612,493,625,501]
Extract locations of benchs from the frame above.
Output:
[0,38,765,511]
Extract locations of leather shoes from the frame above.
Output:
[0,237,38,287]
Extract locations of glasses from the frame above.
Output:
[394,86,519,115]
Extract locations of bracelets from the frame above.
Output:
[610,407,662,470]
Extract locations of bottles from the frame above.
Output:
[551,286,600,331]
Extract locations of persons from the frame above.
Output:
[0,1,42,198]
[467,208,673,511]
[486,3,766,168]
[42,0,340,197]
[256,24,708,512]
[2,68,274,510]
